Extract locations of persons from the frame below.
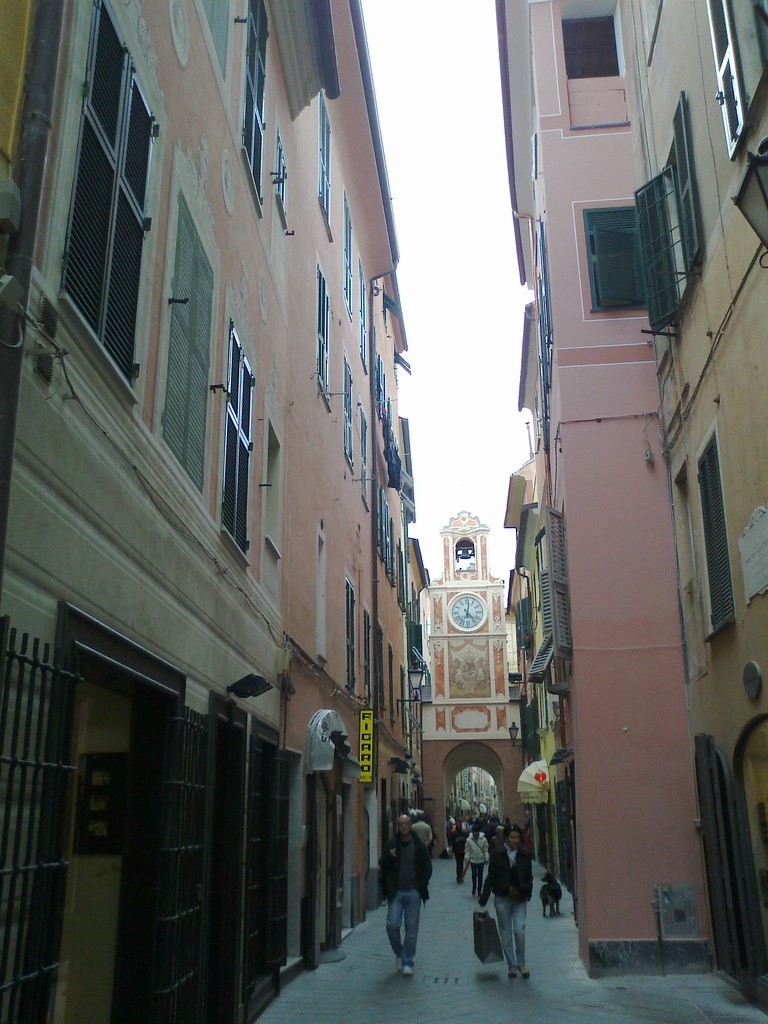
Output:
[411,815,433,850]
[465,826,490,896]
[379,814,433,976]
[479,825,533,979]
[446,812,533,883]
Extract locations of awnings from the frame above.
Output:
[517,759,550,791]
[479,804,487,813]
[461,799,471,810]
[519,791,548,804]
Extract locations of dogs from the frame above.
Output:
[539,871,562,917]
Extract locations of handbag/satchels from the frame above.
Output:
[473,906,504,963]
[508,886,527,902]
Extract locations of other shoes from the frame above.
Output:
[478,887,482,896]
[508,966,517,978]
[402,964,412,975]
[396,956,403,971]
[518,966,530,978]
[472,886,476,895]
[457,877,464,883]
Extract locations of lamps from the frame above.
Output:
[395,761,410,768]
[507,722,525,748]
[387,757,402,765]
[508,673,522,682]
[392,768,408,774]
[396,660,424,717]
[225,673,274,698]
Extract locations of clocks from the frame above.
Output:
[452,597,483,628]
